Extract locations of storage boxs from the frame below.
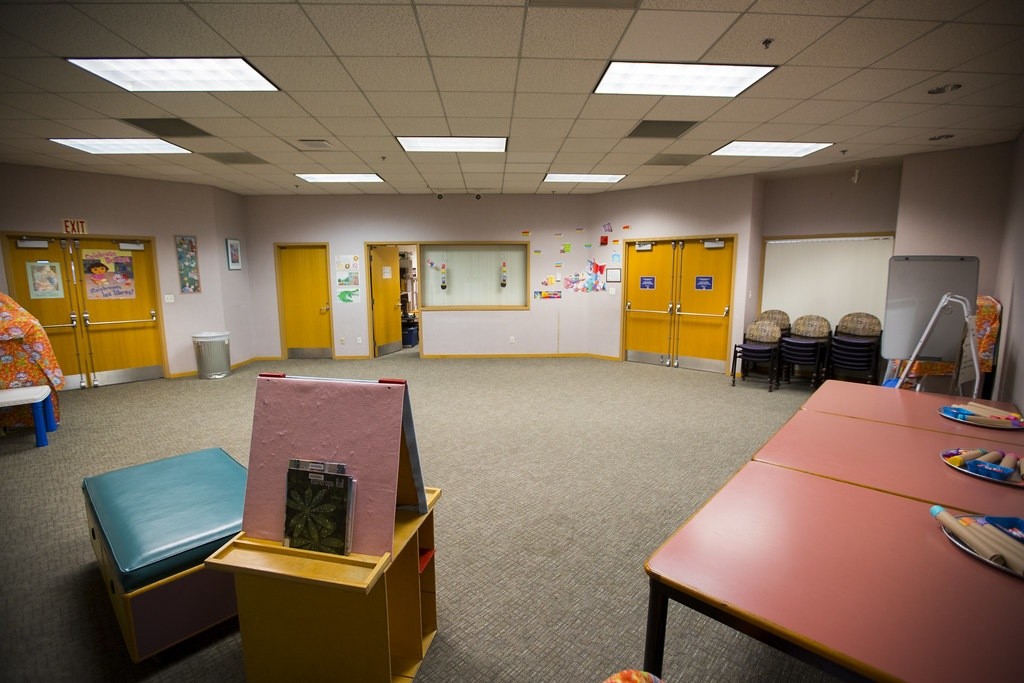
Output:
[402,326,419,348]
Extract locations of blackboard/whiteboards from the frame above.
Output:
[879,253,982,361]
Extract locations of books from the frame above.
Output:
[281,456,356,555]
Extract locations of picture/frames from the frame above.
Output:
[174,235,202,293]
[227,239,242,269]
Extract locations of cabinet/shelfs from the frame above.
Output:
[204,488,443,683]
[399,250,419,347]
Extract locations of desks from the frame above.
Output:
[643,461,1024,683]
[800,379,1024,446]
[0,384,57,447]
[751,410,1024,519]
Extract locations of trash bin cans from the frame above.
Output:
[192,330,232,379]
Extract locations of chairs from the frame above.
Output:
[730,310,884,391]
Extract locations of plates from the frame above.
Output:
[941,515,1024,577]
[937,406,1024,428]
[940,448,1024,486]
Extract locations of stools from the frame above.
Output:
[80,447,249,664]
